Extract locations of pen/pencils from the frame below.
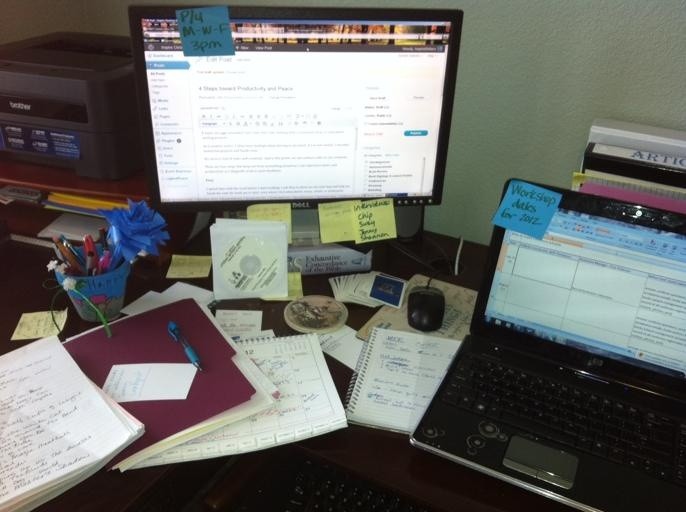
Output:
[52,227,122,277]
[168,320,203,371]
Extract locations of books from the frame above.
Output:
[0,334,149,512]
[128,331,349,472]
[60,296,283,476]
[342,326,462,437]
[0,186,143,254]
[566,118,686,218]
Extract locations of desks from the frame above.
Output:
[1,230,583,512]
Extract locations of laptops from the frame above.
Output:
[406,176,686,512]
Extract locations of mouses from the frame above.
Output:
[404,284,447,332]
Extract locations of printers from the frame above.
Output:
[0,30,149,183]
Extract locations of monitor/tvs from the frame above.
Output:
[126,1,465,248]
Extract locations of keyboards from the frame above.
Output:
[287,454,438,511]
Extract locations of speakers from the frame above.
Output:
[392,205,426,245]
